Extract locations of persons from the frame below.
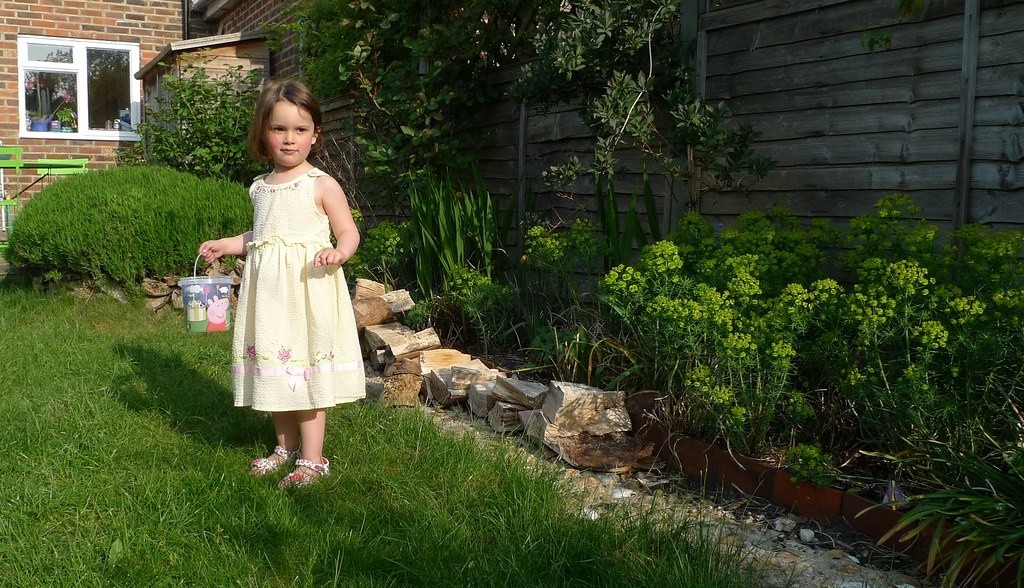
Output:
[198,78,360,490]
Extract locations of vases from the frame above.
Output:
[31,123,49,132]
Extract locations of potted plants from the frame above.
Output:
[56,108,74,127]
[599,193,1024,588]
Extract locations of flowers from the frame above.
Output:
[52,81,76,104]
[29,110,55,123]
[24,71,37,96]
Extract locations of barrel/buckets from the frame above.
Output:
[178,251,234,333]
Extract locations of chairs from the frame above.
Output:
[0,147,89,249]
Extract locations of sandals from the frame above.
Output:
[248,443,302,479]
[282,456,329,488]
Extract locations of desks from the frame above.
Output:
[23,161,84,189]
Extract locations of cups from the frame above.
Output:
[50,120,61,131]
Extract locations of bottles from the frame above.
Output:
[113,119,119,130]
[105,120,112,131]
